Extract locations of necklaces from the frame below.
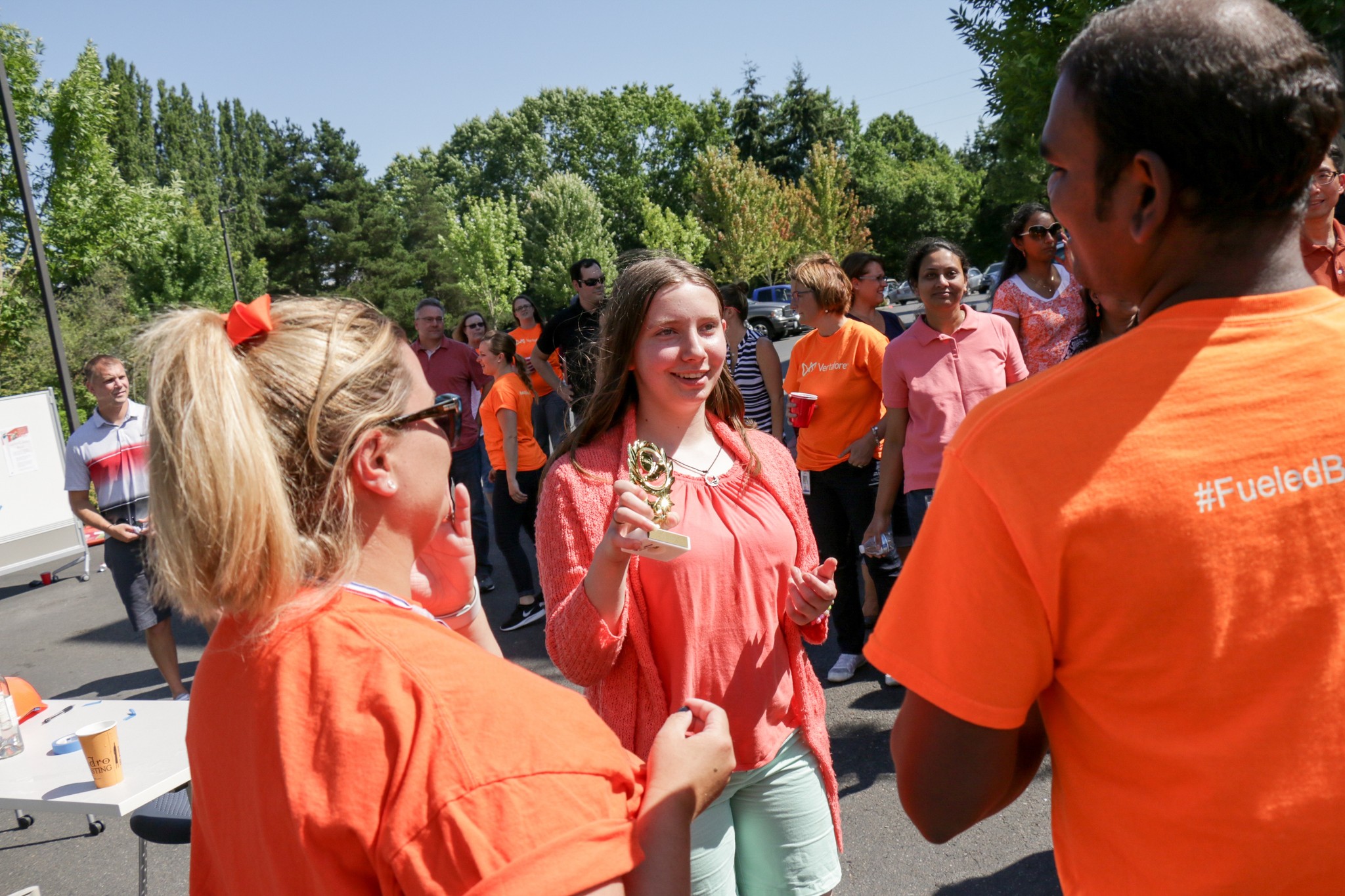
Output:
[1024,267,1054,292]
[659,439,724,486]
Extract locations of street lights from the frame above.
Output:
[217,206,241,300]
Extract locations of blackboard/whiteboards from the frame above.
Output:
[0,387,80,545]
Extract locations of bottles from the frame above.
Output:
[859,531,895,555]
[0,675,24,759]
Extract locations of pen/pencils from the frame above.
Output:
[42,704,75,724]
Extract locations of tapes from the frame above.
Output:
[52,733,82,755]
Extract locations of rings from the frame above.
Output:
[858,465,863,468]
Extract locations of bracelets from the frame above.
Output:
[871,427,881,449]
[432,577,479,622]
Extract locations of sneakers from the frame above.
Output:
[499,602,545,631]
[827,653,869,681]
[885,673,901,686]
[477,575,495,592]
[535,591,547,607]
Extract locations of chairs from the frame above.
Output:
[129,780,195,896]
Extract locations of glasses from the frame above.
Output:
[858,274,888,286]
[416,315,446,323]
[351,394,462,453]
[1308,170,1338,185]
[790,289,813,301]
[576,275,606,286]
[1018,223,1063,241]
[512,304,532,310]
[467,322,485,328]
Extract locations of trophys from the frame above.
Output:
[615,438,691,561]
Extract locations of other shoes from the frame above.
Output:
[862,614,879,625]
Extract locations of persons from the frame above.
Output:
[864,0,1343,896]
[65,148,1344,896]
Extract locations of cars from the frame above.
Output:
[882,279,924,305]
[963,266,983,296]
[747,297,805,342]
[978,260,1006,295]
[752,284,793,303]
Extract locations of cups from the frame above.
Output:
[791,392,818,428]
[41,572,51,585]
[525,357,536,372]
[99,563,107,572]
[75,720,124,788]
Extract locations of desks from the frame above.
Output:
[0,695,194,817]
